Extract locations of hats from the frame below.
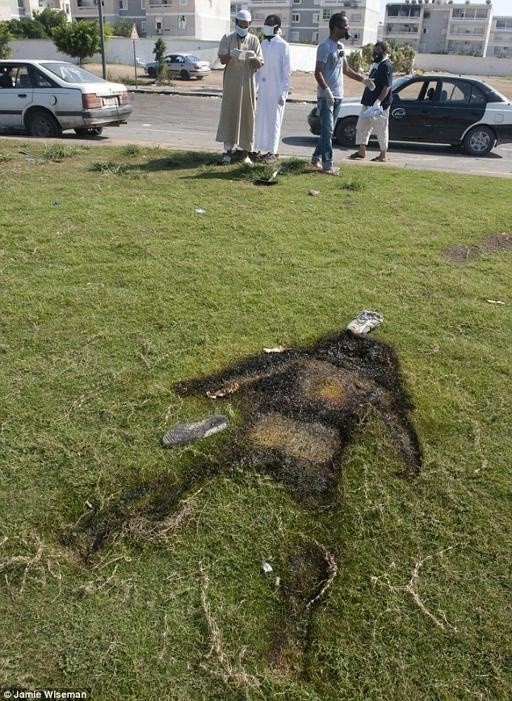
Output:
[236,10,252,22]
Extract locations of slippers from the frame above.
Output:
[351,152,365,158]
[371,157,386,162]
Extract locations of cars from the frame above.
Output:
[307,70,512,156]
[144,52,210,80]
[0,58,133,137]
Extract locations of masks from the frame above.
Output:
[372,50,385,62]
[262,24,278,36]
[235,25,250,37]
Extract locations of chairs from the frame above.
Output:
[424,88,447,102]
[1,74,30,88]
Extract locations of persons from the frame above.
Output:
[254,15,291,163]
[311,12,376,174]
[346,41,393,161]
[216,10,265,165]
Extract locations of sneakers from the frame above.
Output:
[163,412,229,449]
[222,153,231,164]
[312,159,336,173]
[348,310,385,335]
[251,152,279,163]
[242,150,253,158]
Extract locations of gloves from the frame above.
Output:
[362,77,376,92]
[325,87,334,106]
[230,48,241,57]
[246,51,257,60]
